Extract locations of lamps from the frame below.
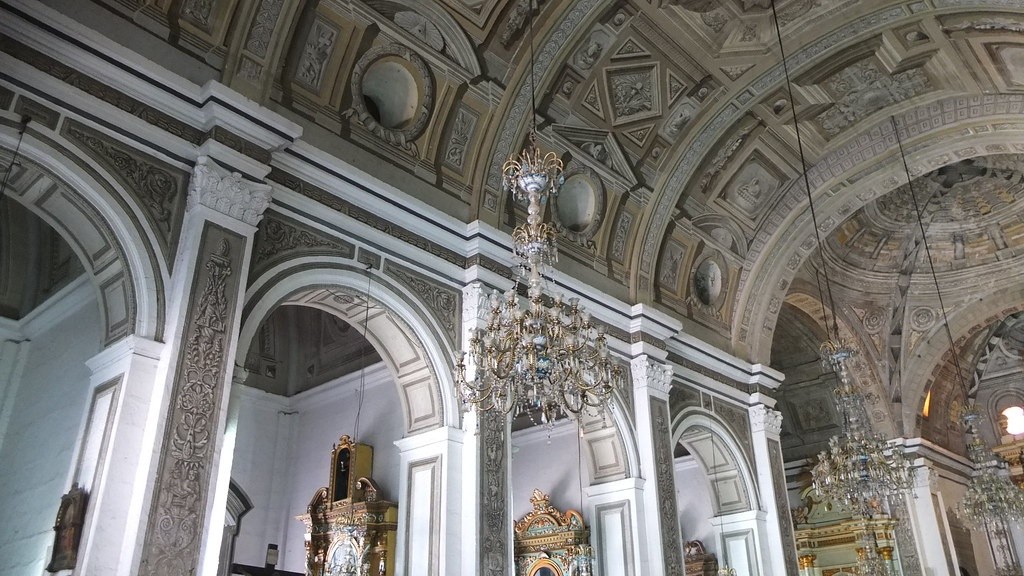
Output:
[768,1,923,521]
[889,115,1023,536]
[447,0,629,429]
